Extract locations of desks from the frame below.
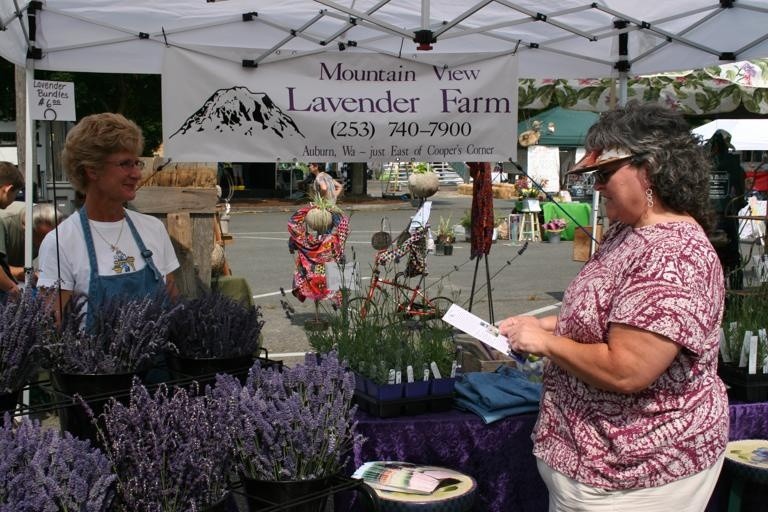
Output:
[516,201,591,240]
[316,352,768,512]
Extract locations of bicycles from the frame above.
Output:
[341,248,461,332]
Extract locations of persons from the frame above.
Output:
[0,198,66,289]
[1,160,22,424]
[496,97,732,512]
[308,162,345,207]
[34,112,190,380]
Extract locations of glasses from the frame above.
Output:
[104,158,145,170]
[592,158,638,185]
[306,164,314,168]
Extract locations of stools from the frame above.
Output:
[725,437,768,512]
[371,465,477,512]
[519,212,541,241]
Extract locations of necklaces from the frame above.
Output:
[84,206,127,254]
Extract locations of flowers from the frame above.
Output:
[514,175,547,199]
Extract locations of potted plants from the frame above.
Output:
[306,190,333,230]
[409,161,439,198]
[719,256,768,403]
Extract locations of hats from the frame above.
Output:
[563,142,646,176]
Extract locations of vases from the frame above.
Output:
[520,199,541,212]
